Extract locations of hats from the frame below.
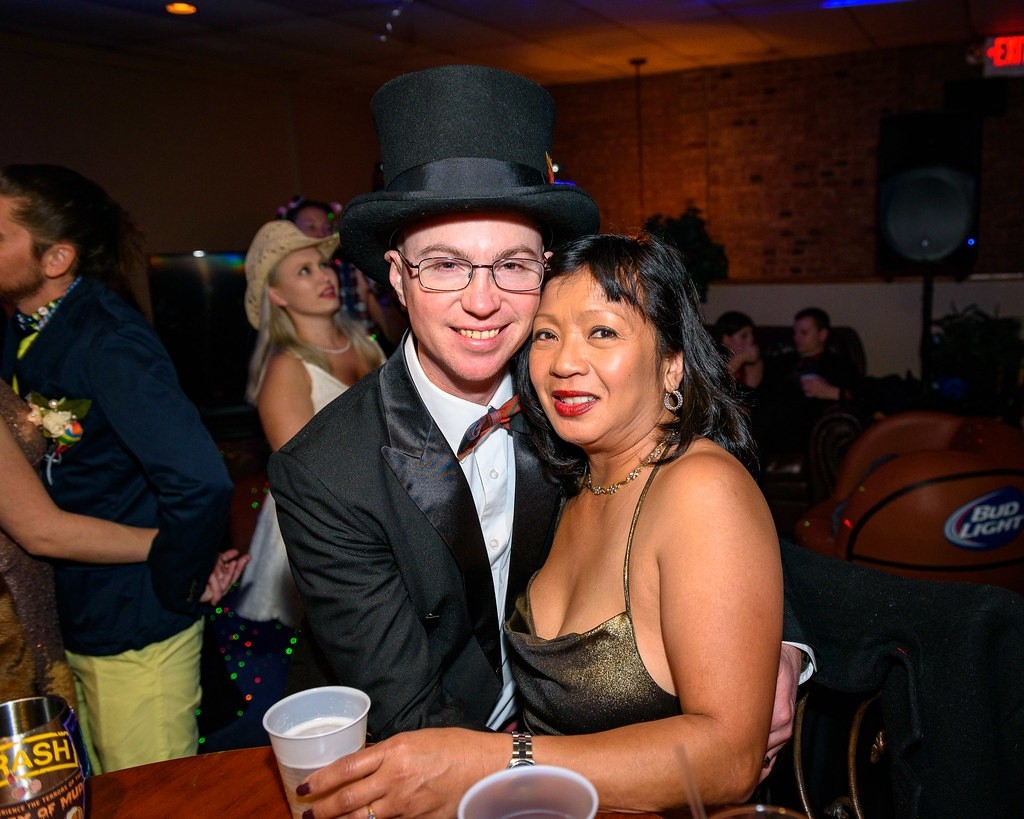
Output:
[245,220,337,332]
[338,64,603,287]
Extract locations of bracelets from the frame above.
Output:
[506,730,535,769]
[839,387,848,401]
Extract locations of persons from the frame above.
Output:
[266,64,819,784]
[0,163,396,777]
[710,306,863,485]
[294,235,786,819]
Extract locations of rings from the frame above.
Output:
[368,805,376,819]
[763,755,771,765]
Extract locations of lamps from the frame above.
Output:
[869,105,983,380]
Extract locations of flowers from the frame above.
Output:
[26,392,89,462]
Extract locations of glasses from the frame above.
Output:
[395,248,551,292]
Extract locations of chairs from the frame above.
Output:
[705,627,926,819]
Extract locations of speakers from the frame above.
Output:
[872,109,987,284]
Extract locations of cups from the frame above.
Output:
[0,695,93,819]
[801,374,818,381]
[262,685,371,819]
[457,764,600,818]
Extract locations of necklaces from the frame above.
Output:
[316,339,352,354]
[586,432,678,496]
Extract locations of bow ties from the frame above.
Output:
[457,395,520,462]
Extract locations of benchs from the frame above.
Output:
[707,324,866,518]
